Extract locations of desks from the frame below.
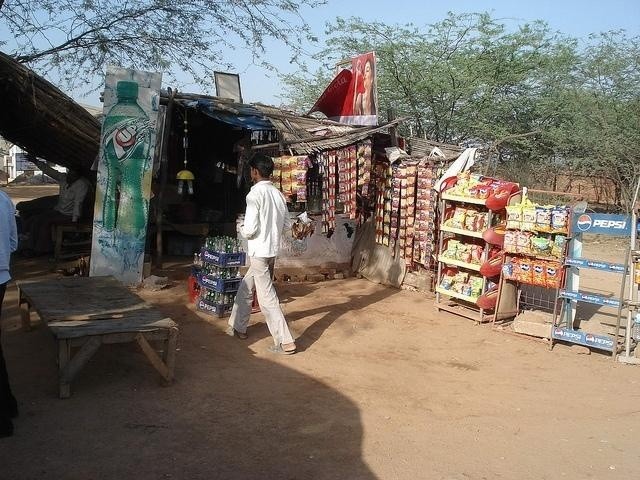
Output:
[14,275,181,400]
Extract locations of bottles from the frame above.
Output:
[633,309,640,341]
[194,236,243,312]
[79,255,86,277]
[103,81,151,234]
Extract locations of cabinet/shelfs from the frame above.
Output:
[435,185,583,346]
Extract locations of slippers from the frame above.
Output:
[267,343,296,354]
[234,329,248,339]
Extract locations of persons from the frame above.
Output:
[21,153,90,256]
[226,152,297,356]
[354,58,376,115]
[0,189,19,438]
